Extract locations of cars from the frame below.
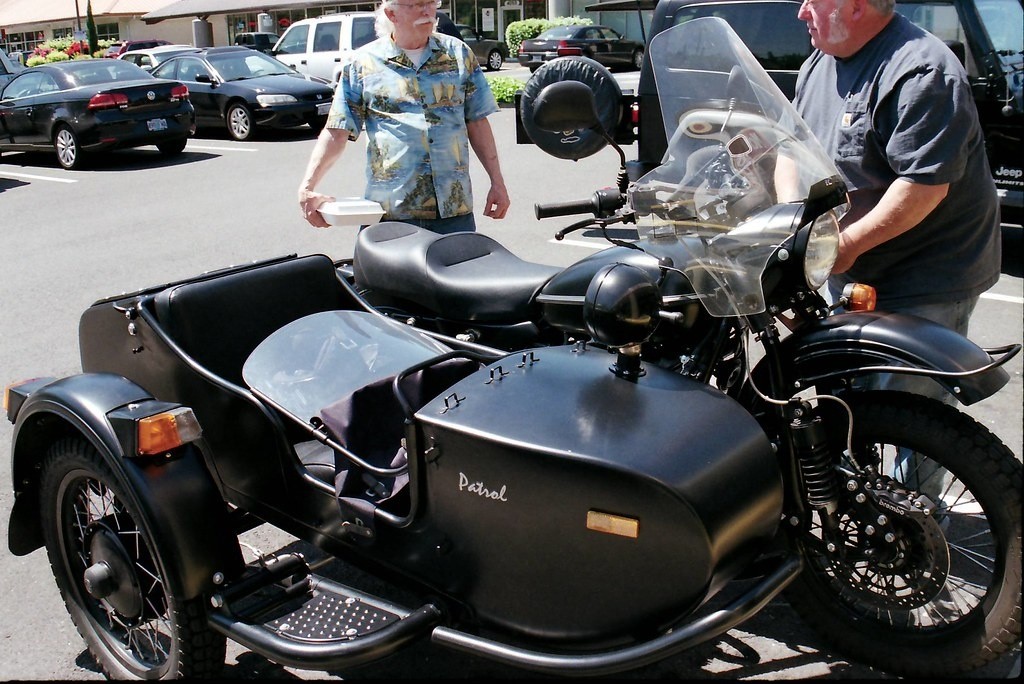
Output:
[519,24,644,71]
[453,23,508,71]
[0,59,195,170]
[147,46,335,141]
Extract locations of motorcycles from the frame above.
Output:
[6,15,1024,684]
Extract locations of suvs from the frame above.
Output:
[104,39,174,59]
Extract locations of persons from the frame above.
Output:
[773,0,1001,534]
[297,0,509,234]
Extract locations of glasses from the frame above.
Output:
[390,0,442,10]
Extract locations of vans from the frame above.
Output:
[0,47,42,100]
[587,0,1024,232]
[272,13,378,84]
[233,33,280,55]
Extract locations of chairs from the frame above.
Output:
[319,34,337,52]
[154,253,373,389]
[115,69,135,80]
[78,73,105,86]
[187,65,204,80]
